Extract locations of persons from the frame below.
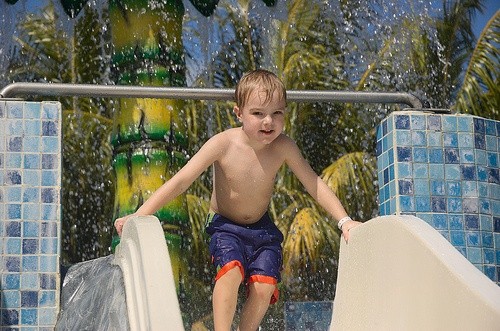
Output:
[110,69,365,331]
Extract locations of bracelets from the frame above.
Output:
[337,216,352,231]
